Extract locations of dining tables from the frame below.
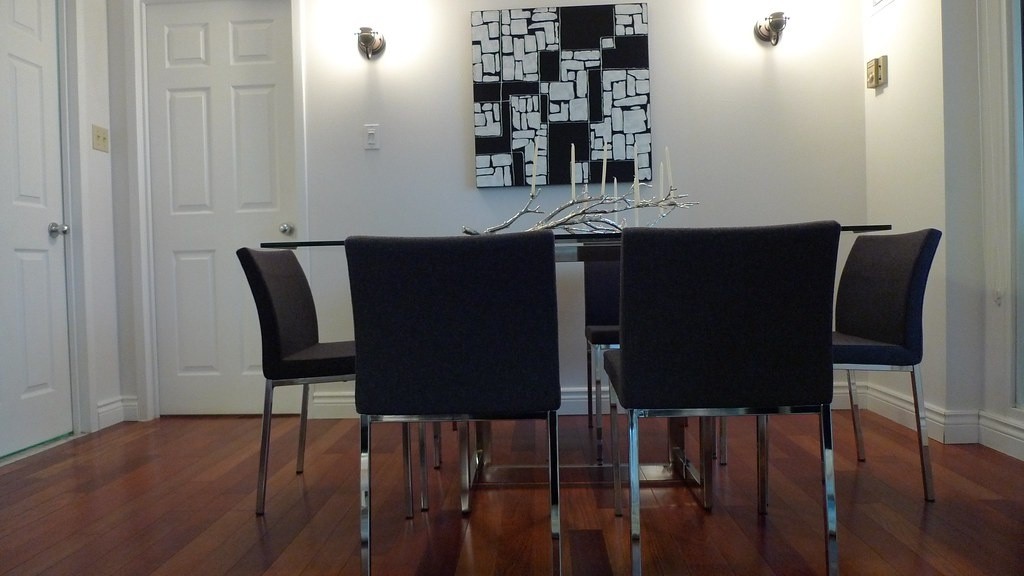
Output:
[259,223,897,520]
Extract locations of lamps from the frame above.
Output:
[754,12,791,47]
[353,26,388,59]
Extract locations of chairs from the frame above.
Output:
[235,247,417,522]
[602,219,842,545]
[830,227,944,503]
[343,229,564,542]
